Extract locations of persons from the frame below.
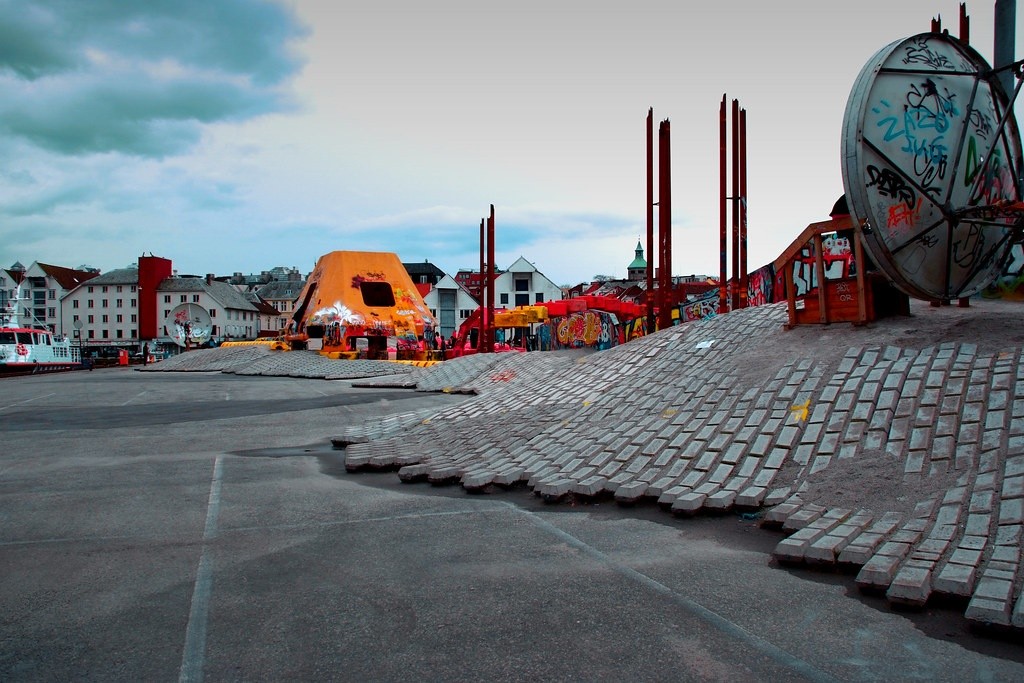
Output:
[142,342,151,366]
[495,327,505,343]
[434,331,455,349]
